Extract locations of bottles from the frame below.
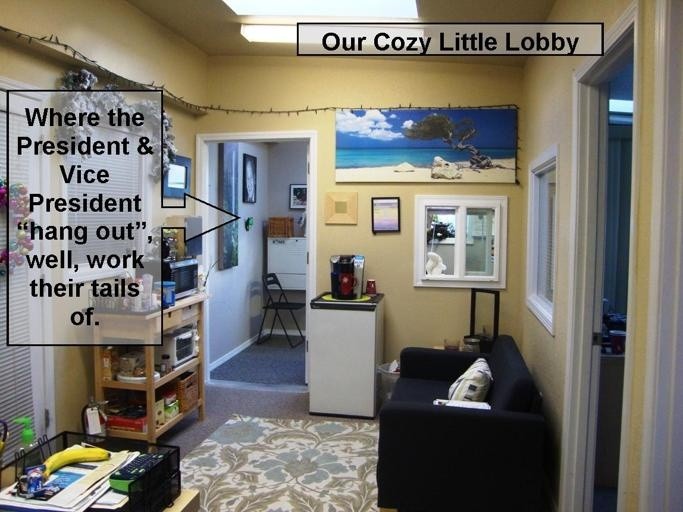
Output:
[160,354,171,378]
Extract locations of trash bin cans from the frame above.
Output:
[377,362,401,409]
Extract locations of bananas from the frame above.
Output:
[42,448,110,480]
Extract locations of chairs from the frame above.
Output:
[257,273,305,348]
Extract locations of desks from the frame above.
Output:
[0,465,199,512]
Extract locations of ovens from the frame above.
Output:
[152,327,201,368]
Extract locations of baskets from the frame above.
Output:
[132,370,200,416]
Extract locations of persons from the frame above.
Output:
[245,158,255,203]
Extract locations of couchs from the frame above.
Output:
[376,335,545,511]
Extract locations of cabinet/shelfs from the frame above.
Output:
[310,292,384,419]
[82,292,212,444]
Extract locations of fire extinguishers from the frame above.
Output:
[80,396,110,450]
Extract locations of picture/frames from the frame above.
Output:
[243,153,257,203]
[163,155,192,199]
[290,184,307,209]
[372,198,400,233]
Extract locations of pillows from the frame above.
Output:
[433,357,494,410]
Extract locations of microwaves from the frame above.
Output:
[132,256,200,301]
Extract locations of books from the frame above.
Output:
[265,216,295,238]
[0,441,142,512]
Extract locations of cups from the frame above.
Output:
[366,278,377,297]
[339,272,358,296]
[87,288,124,310]
[609,330,626,355]
